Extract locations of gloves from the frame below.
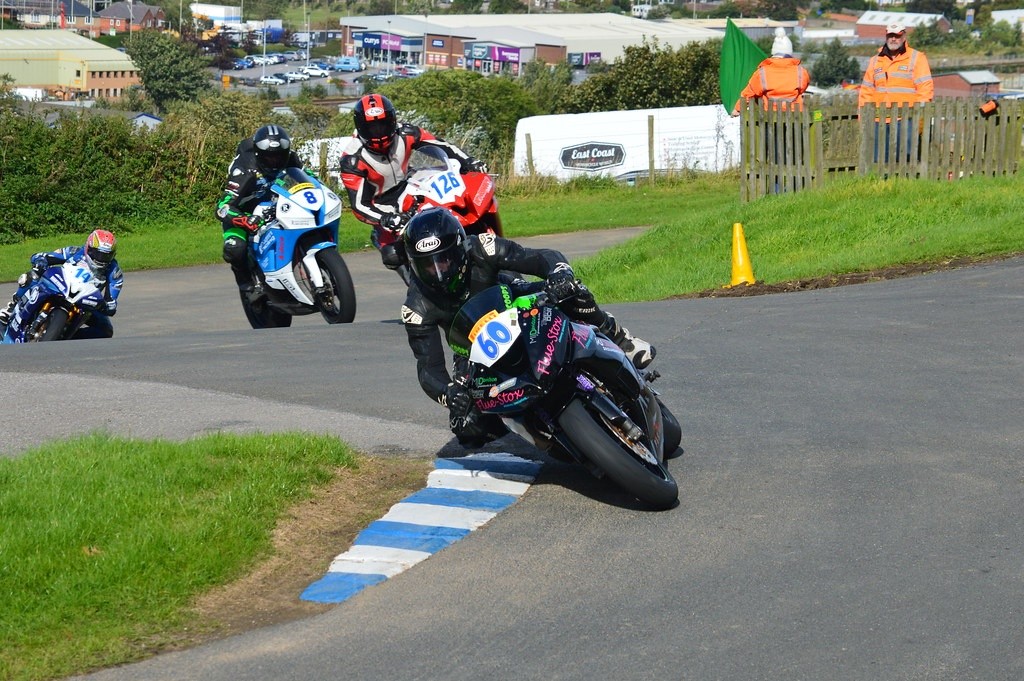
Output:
[381,212,402,230]
[545,263,577,304]
[34,255,48,270]
[436,382,471,418]
[232,212,265,231]
[463,157,490,174]
[106,298,117,311]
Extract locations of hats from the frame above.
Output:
[886,22,907,35]
[771,27,793,58]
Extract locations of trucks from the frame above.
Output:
[285,32,316,48]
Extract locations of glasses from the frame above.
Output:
[419,248,454,269]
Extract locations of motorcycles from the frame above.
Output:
[369,145,504,286]
[0,266,116,344]
[240,167,356,329]
[449,280,681,511]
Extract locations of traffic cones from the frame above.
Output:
[723,223,756,288]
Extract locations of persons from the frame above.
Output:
[0,230,123,340]
[401,207,657,450]
[216,126,324,306]
[858,22,934,163]
[731,27,810,193]
[339,94,489,286]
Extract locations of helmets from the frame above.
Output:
[84,229,117,271]
[353,93,397,157]
[402,206,471,302]
[253,125,290,177]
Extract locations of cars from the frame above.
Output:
[232,49,312,71]
[260,56,367,86]
[353,65,424,84]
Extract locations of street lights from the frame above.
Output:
[387,20,391,75]
[306,12,310,67]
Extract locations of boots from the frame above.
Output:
[598,310,656,370]
[230,266,254,293]
[0,292,21,325]
